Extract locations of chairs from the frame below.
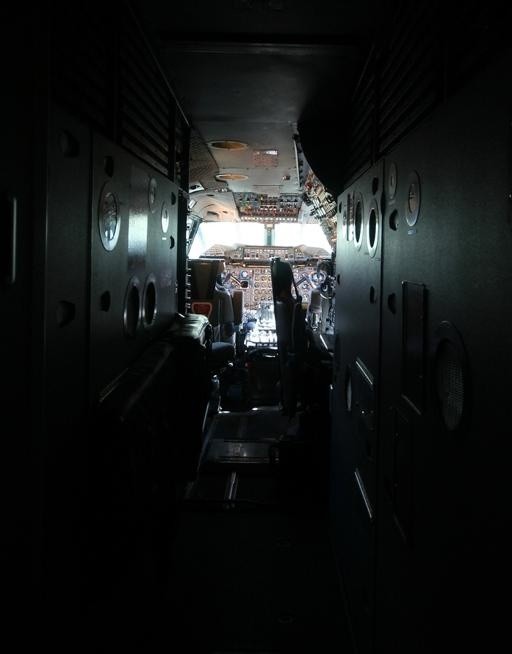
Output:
[271,258,315,369]
[190,261,233,366]
